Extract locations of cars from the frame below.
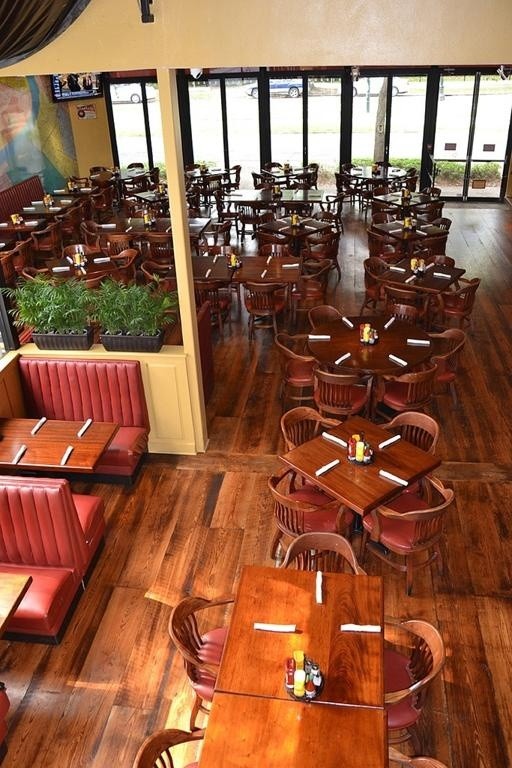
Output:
[335,77,411,98]
[109,83,159,106]
[244,78,313,98]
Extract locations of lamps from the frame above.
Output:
[188,67,204,82]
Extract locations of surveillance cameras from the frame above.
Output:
[190,68,204,80]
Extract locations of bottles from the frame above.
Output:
[359,323,379,345]
[67,181,79,192]
[347,431,375,464]
[143,213,157,227]
[403,215,417,232]
[271,185,281,195]
[290,214,301,227]
[43,194,54,207]
[10,214,24,225]
[401,188,412,199]
[411,256,427,276]
[72,251,87,268]
[283,649,323,701]
[227,252,243,270]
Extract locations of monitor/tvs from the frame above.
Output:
[49,72,105,103]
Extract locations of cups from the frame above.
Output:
[159,185,165,195]
